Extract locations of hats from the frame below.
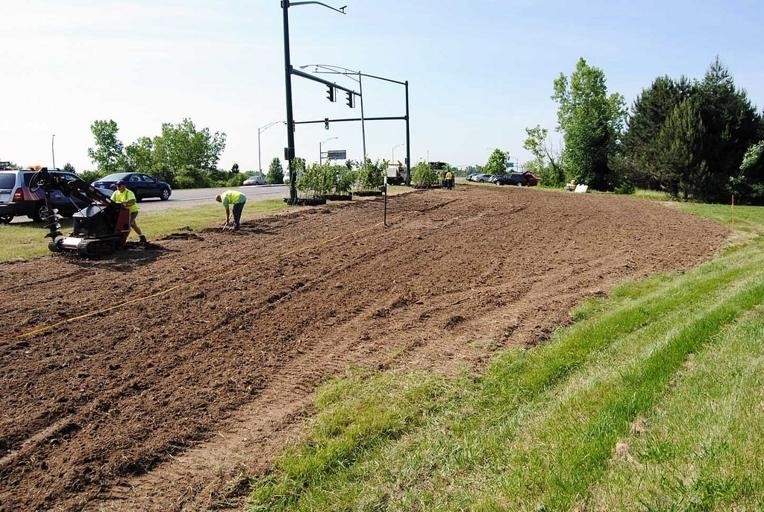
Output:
[115,180,125,185]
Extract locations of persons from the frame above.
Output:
[109,179,147,243]
[215,190,246,231]
[436,171,443,187]
[445,170,453,190]
[31,165,61,229]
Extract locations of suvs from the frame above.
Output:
[0,169,115,225]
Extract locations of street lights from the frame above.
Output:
[51,134,56,168]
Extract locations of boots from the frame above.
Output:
[229,220,240,230]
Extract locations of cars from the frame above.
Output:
[466,172,539,187]
[242,175,264,186]
[90,172,171,202]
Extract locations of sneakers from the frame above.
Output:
[140,236,146,242]
